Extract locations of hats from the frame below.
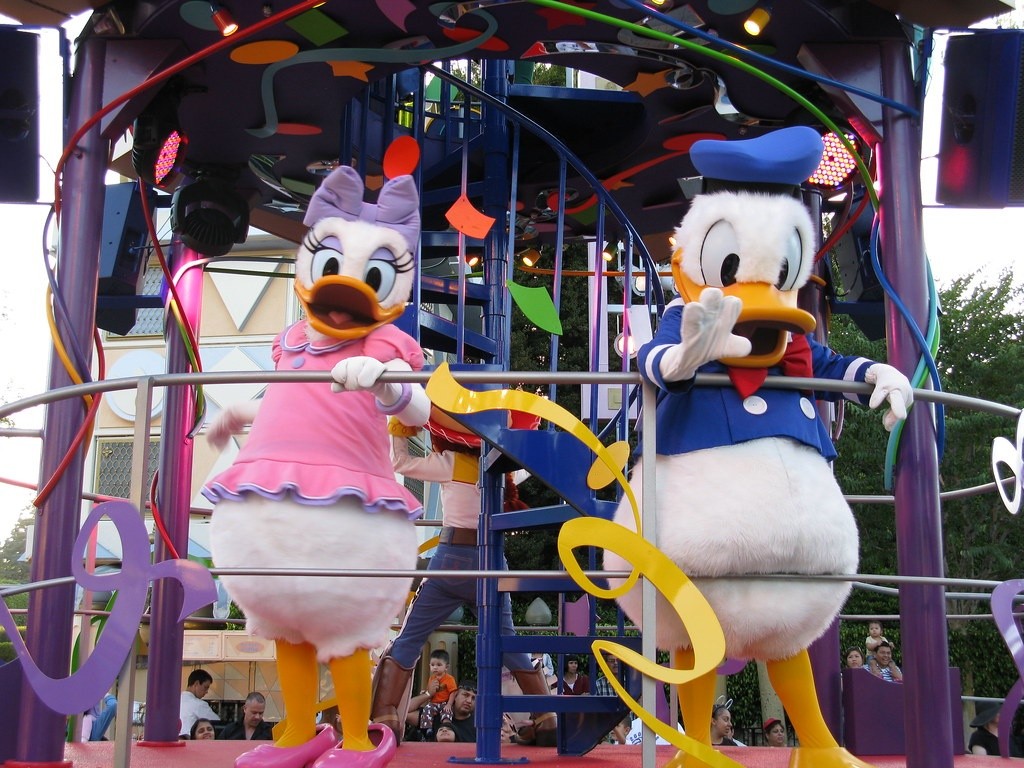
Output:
[762,718,781,730]
[969,699,1003,728]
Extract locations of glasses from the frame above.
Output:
[457,685,478,694]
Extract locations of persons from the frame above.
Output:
[215,691,273,740]
[178,669,220,736]
[80,691,117,742]
[841,622,903,697]
[190,718,215,740]
[563,655,591,695]
[600,697,685,746]
[595,652,627,697]
[369,401,561,746]
[968,699,1020,757]
[762,718,790,747]
[710,703,747,746]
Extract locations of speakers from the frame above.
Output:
[95,181,158,337]
[0,29,39,205]
[934,30,1024,208]
[832,191,887,340]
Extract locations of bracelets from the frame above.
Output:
[425,689,431,698]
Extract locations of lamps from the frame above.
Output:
[783,99,865,192]
[170,176,251,257]
[206,0,241,37]
[744,0,776,36]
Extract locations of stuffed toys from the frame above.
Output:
[203,165,433,767]
[600,125,913,768]
